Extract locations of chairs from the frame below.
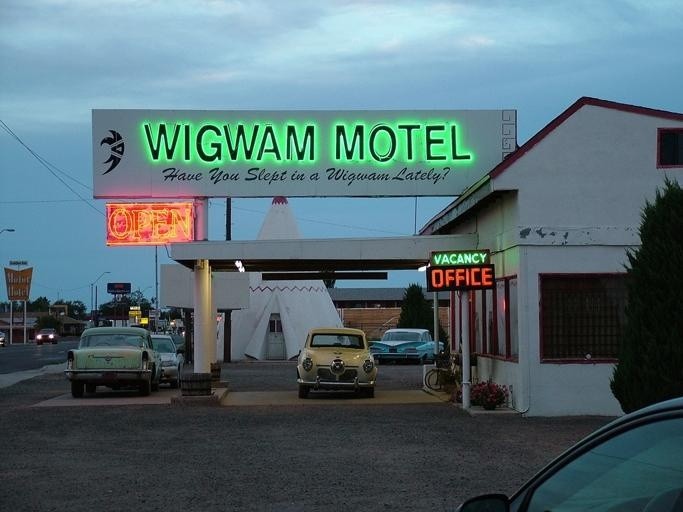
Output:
[154,342,171,353]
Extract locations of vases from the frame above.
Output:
[483,403,496,409]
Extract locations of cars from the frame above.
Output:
[64,326,185,397]
[35,328,58,345]
[296,328,444,398]
[0,332,8,346]
[453,397,682,511]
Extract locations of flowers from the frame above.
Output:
[450,380,509,408]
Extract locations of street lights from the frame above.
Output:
[91,272,110,309]
[0,228,15,235]
